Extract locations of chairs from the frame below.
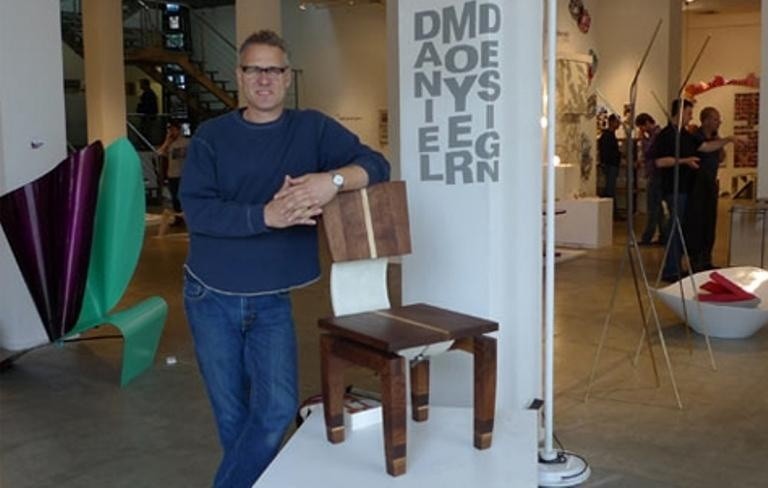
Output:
[313,179,501,477]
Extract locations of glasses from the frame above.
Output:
[239,66,289,76]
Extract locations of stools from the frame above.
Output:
[649,265,767,341]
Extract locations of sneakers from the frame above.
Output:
[652,239,668,245]
[662,270,689,284]
[168,222,185,228]
[630,240,651,247]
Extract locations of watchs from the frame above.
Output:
[327,169,347,194]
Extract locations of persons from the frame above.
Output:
[179,29,396,487]
[593,97,752,282]
[155,118,193,229]
[136,76,159,150]
